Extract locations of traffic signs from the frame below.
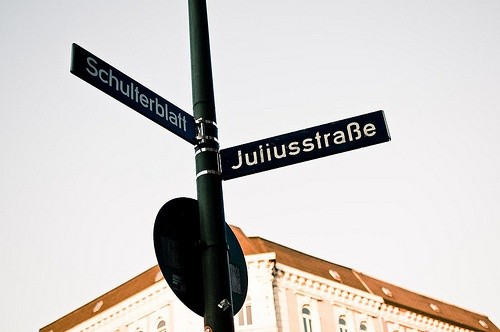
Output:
[68,41,202,149]
[219,110,392,187]
